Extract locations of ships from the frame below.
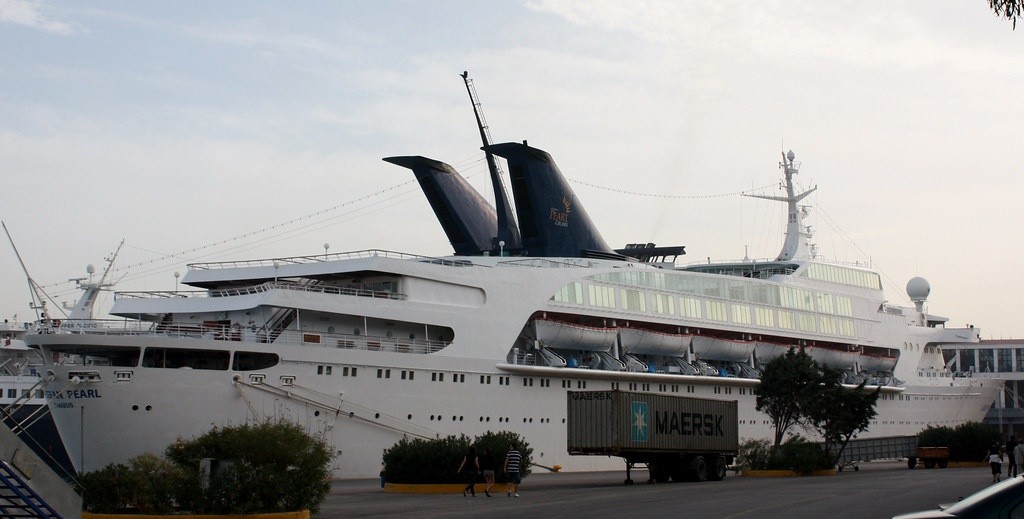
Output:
[0,68,1007,487]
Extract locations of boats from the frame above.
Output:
[689,333,757,363]
[859,354,897,373]
[802,344,861,371]
[613,242,687,256]
[531,314,620,353]
[617,326,693,358]
[753,339,801,365]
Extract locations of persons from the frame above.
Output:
[504,444,523,497]
[989,441,1004,482]
[1006,435,1024,477]
[457,447,495,498]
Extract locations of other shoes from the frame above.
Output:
[507,491,511,497]
[514,492,519,497]
[463,489,466,496]
[485,489,489,493]
[486,494,492,497]
[472,495,477,497]
